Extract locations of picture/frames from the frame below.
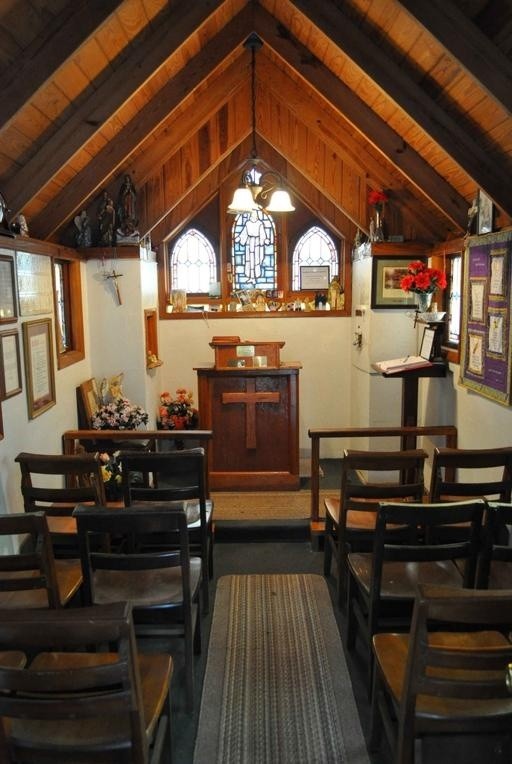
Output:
[0,245,57,446]
[475,186,494,236]
[79,376,104,447]
[370,254,428,310]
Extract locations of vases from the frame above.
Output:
[415,292,431,313]
[374,210,387,244]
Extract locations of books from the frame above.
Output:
[372,355,432,375]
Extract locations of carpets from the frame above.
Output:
[192,571,376,764]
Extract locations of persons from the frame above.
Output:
[73,174,137,248]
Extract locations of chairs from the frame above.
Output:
[0,447,220,763]
[323,444,512,764]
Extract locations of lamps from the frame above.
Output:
[223,29,296,213]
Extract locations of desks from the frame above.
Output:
[370,354,450,485]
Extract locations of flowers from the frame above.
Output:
[364,184,392,211]
[396,258,448,305]
[90,371,198,487]
